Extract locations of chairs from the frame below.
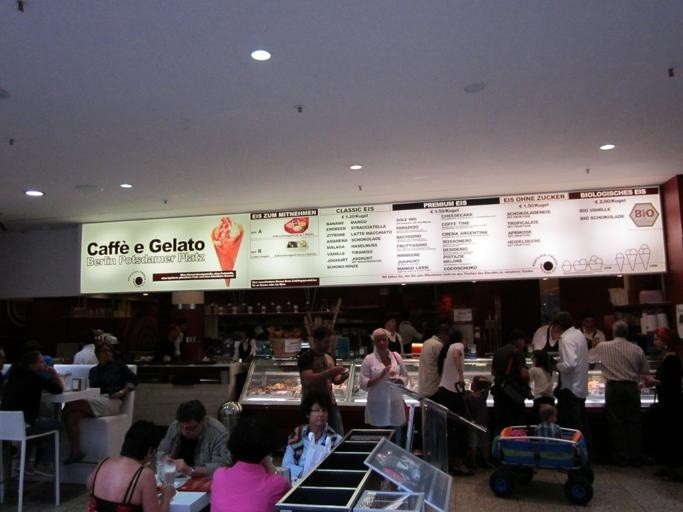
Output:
[0,411,62,512]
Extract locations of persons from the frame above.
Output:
[62,341,140,428]
[85,418,177,512]
[530,321,559,354]
[73,336,99,364]
[296,326,350,435]
[2,351,66,478]
[416,323,454,460]
[491,329,529,431]
[534,403,563,440]
[587,319,650,466]
[381,314,405,356]
[554,310,590,427]
[281,390,344,485]
[438,330,475,477]
[174,318,188,357]
[397,319,426,354]
[643,325,683,481]
[0,348,9,401]
[209,414,293,512]
[358,326,410,449]
[157,398,233,480]
[233,329,257,362]
[465,374,492,469]
[577,315,606,370]
[26,339,54,368]
[527,348,557,422]
[150,324,182,360]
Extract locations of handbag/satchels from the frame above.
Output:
[403,400,410,419]
[500,348,532,406]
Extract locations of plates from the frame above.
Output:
[639,290,662,303]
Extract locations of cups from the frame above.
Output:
[164,463,177,484]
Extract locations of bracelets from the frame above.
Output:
[190,467,197,476]
[119,389,125,396]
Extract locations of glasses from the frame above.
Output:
[312,408,327,412]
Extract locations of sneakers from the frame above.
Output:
[15,458,34,475]
[33,464,56,477]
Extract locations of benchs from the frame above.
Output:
[2,359,137,488]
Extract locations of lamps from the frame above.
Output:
[172,290,204,310]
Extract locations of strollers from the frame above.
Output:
[485,418,598,503]
